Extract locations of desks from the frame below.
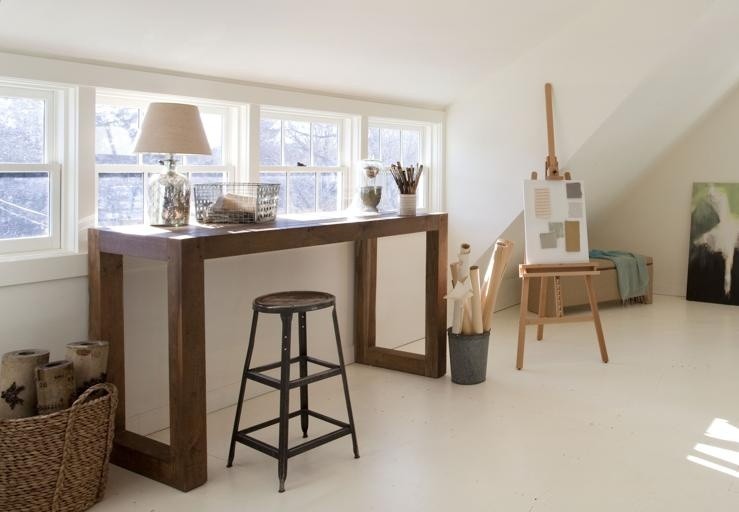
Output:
[87,208,450,492]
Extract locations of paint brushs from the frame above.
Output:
[389,162,424,194]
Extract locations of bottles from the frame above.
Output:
[360,159,383,213]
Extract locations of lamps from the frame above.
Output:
[136,99,213,229]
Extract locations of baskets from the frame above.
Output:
[0,378,121,511]
[192,182,280,224]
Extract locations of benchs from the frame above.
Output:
[528,255,652,317]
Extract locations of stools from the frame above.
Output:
[226,290,360,490]
